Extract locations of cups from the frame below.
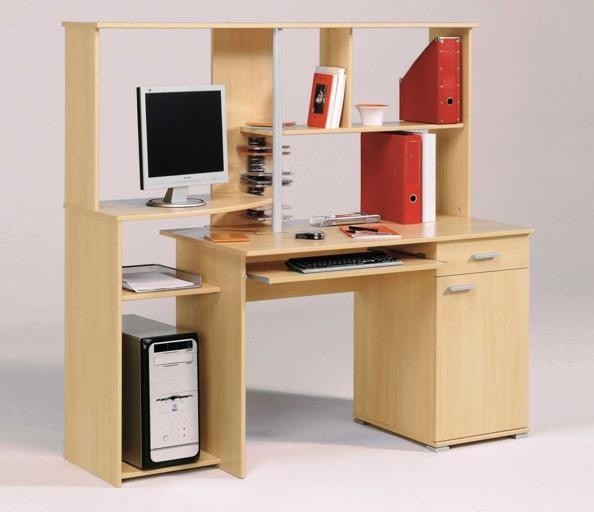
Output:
[354,103,389,126]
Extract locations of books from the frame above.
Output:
[338,223,402,240]
[237,120,294,222]
[307,66,347,129]
[204,231,248,243]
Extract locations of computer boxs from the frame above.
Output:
[122,313,200,471]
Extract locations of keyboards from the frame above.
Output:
[284,251,403,274]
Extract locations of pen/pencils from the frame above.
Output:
[348,226,378,231]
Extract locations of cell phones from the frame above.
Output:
[294,228,326,241]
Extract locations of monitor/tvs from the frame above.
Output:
[137,83,229,208]
